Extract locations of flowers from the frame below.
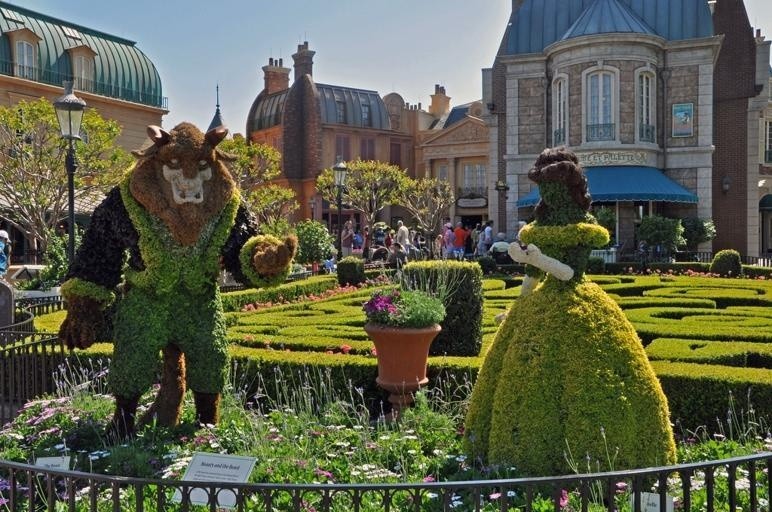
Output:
[361,285,448,329]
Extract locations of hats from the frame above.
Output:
[444,223,453,228]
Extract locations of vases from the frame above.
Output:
[363,319,442,424]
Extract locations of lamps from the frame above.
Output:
[722,173,730,195]
[496,175,510,200]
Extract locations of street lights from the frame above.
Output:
[53,78,86,267]
[332,159,347,261]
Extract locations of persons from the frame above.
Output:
[55,224,65,236]
[331,216,529,264]
[0,230,12,278]
[638,239,666,257]
